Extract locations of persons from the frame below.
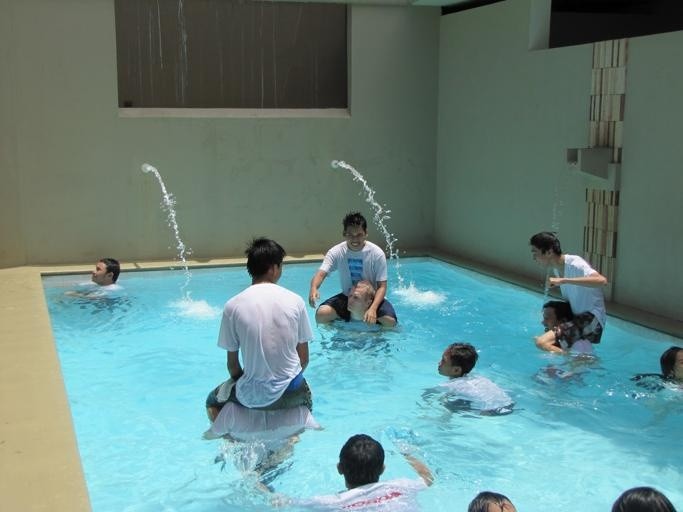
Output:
[322,279,393,360]
[625,346,683,421]
[418,342,515,423]
[307,211,399,348]
[467,491,518,511]
[528,231,608,363]
[204,235,314,478]
[529,301,601,401]
[48,255,126,309]
[197,398,328,499]
[239,433,434,512]
[612,486,679,511]
